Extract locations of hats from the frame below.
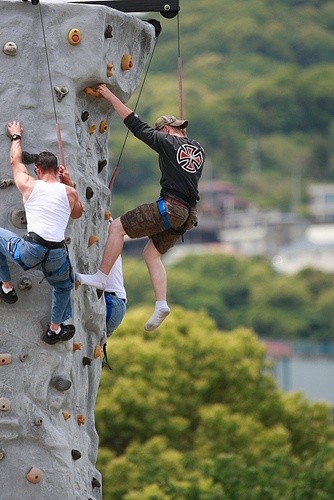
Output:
[155,114,189,131]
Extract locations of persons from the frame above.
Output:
[75,84,204,331]
[102,215,126,338]
[0,119,82,345]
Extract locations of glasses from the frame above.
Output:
[33,164,40,175]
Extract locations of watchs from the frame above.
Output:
[9,134,21,141]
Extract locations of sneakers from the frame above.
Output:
[0,281,18,304]
[43,323,75,345]
